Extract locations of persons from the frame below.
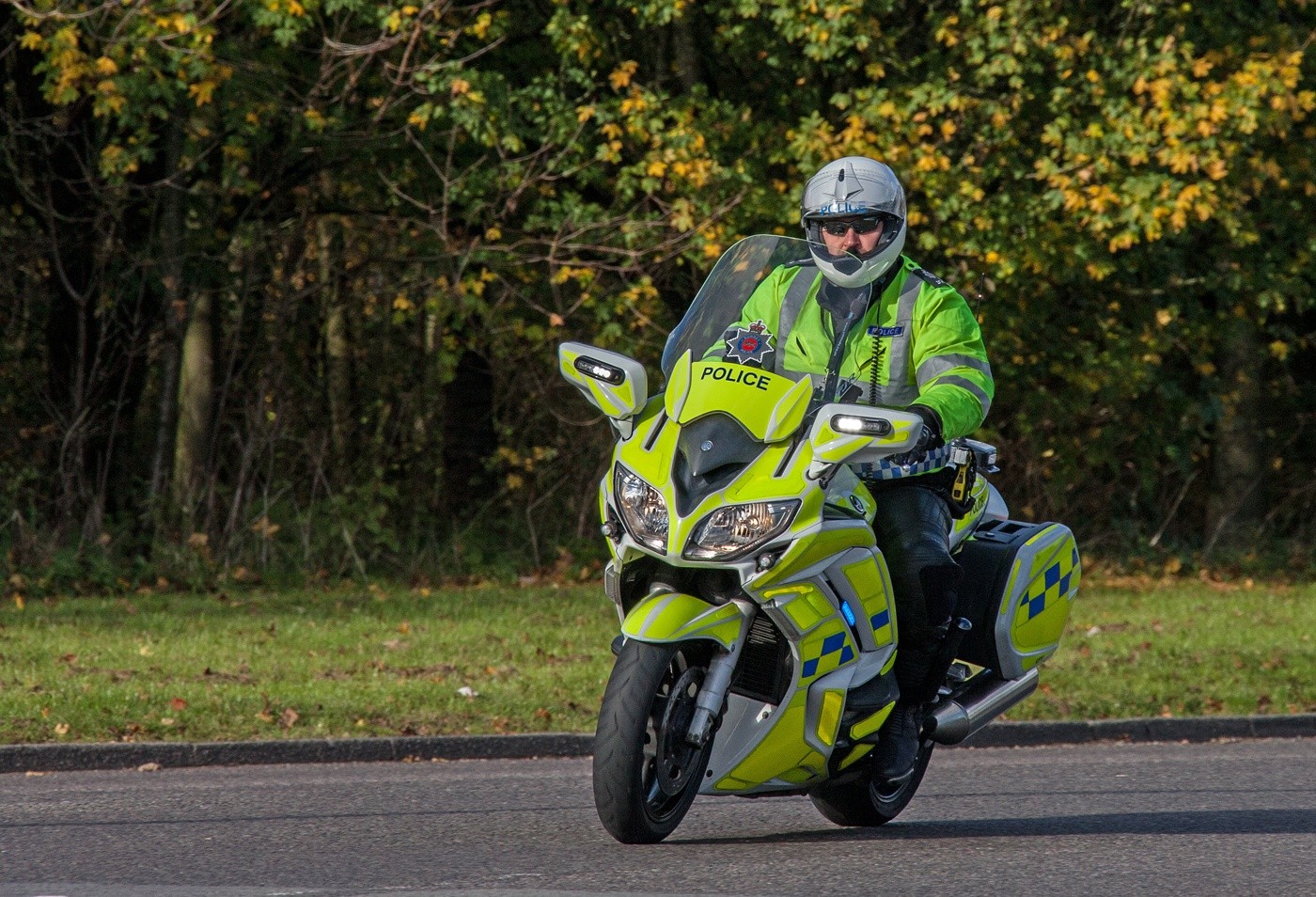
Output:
[699,155,997,785]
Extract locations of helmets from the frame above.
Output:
[801,157,907,288]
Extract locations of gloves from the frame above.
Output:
[885,410,938,466]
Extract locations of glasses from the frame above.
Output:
[819,216,883,235]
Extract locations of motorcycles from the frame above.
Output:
[558,233,1080,845]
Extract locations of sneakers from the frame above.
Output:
[871,698,922,787]
